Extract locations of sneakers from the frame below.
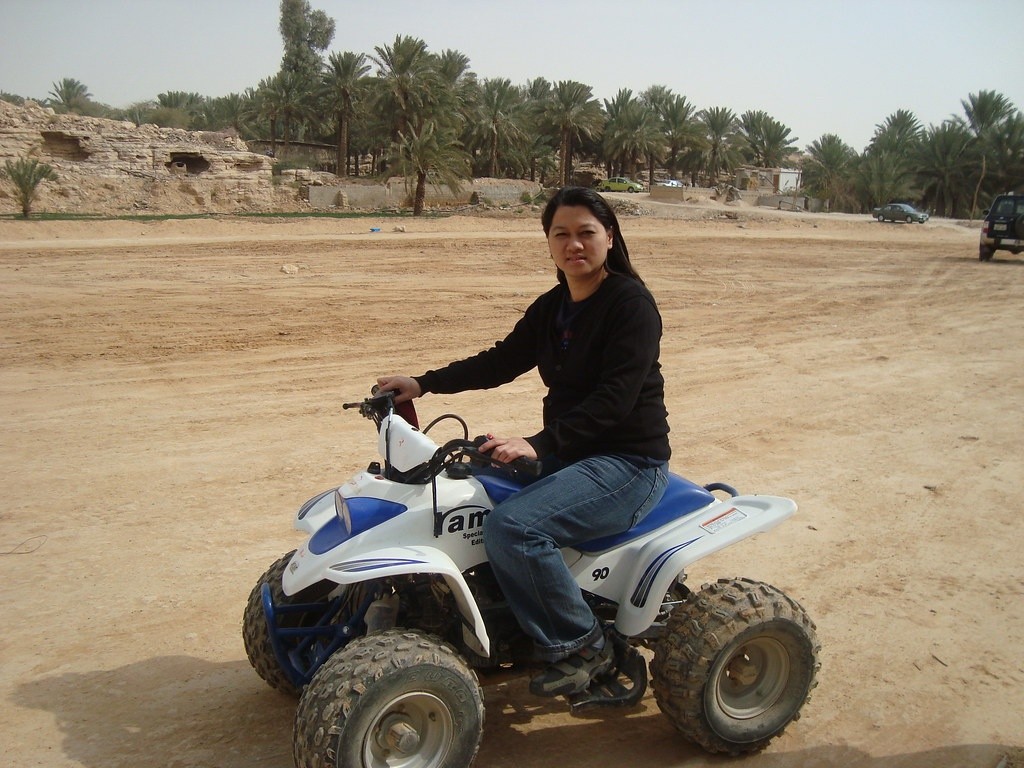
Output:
[528,632,616,701]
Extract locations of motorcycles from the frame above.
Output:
[240,379,830,768]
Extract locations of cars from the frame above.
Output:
[656,180,683,188]
[599,177,643,193]
[871,203,929,224]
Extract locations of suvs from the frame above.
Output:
[977,193,1024,260]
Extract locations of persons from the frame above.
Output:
[377,186,672,698]
